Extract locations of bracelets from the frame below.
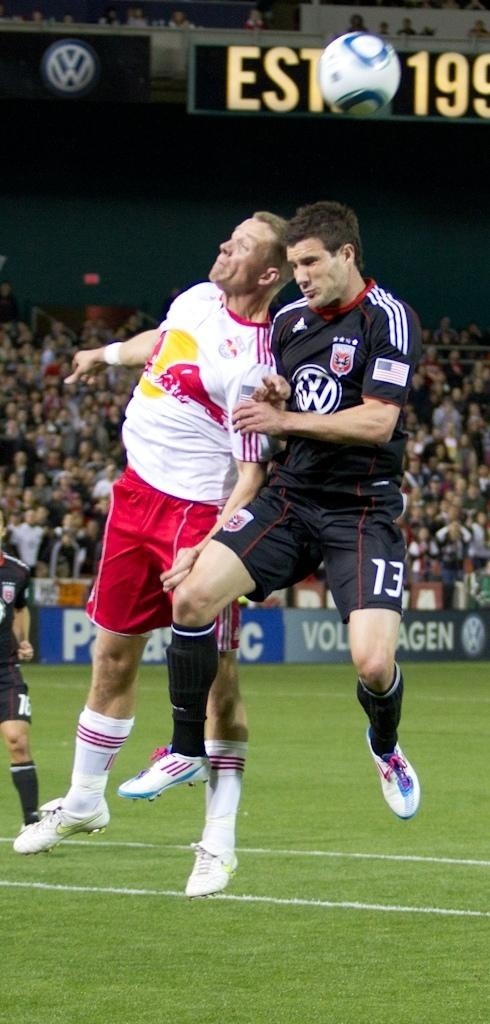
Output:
[104,341,120,367]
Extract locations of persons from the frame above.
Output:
[0,281,189,579]
[0,510,39,833]
[116,200,421,821]
[392,319,490,585]
[11,203,306,897]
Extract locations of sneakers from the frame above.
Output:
[117,749,211,800]
[12,798,110,853]
[184,841,237,897]
[366,723,422,820]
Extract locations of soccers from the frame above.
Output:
[317,31,400,118]
